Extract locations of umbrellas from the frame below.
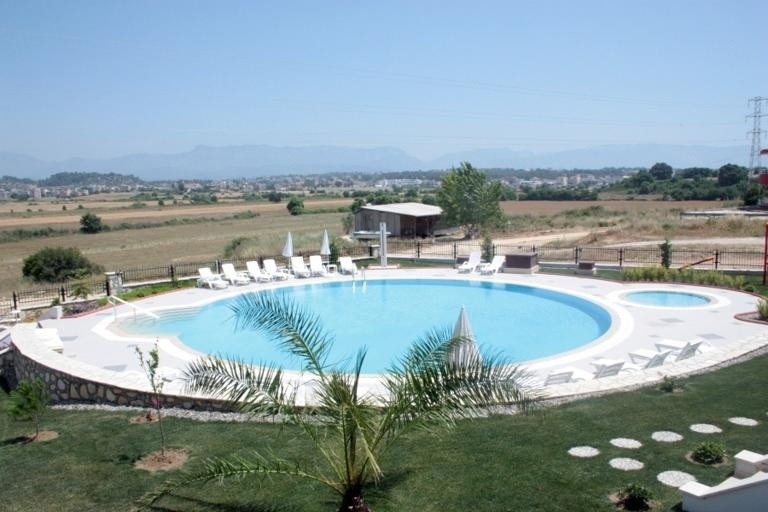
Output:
[320,229,332,272]
[281,231,294,273]
[444,304,483,366]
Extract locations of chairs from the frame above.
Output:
[540,340,705,388]
[457,250,507,276]
[196,256,360,290]
